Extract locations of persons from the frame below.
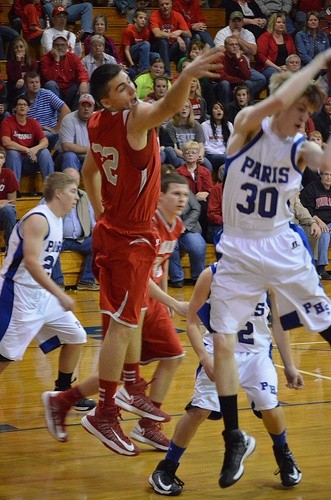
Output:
[146,242,304,496]
[0,0,331,457]
[211,44,331,489]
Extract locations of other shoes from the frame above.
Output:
[77,281,100,290]
[57,284,65,291]
[172,281,183,288]
[320,271,331,279]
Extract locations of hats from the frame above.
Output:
[230,11,243,20]
[78,93,95,107]
[52,33,67,41]
[51,6,68,17]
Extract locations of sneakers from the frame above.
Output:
[80,406,140,457]
[41,391,68,442]
[112,378,170,422]
[130,418,170,450]
[219,428,256,488]
[273,443,302,487]
[148,459,184,495]
[72,398,96,411]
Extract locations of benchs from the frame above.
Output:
[0,1,331,288]
